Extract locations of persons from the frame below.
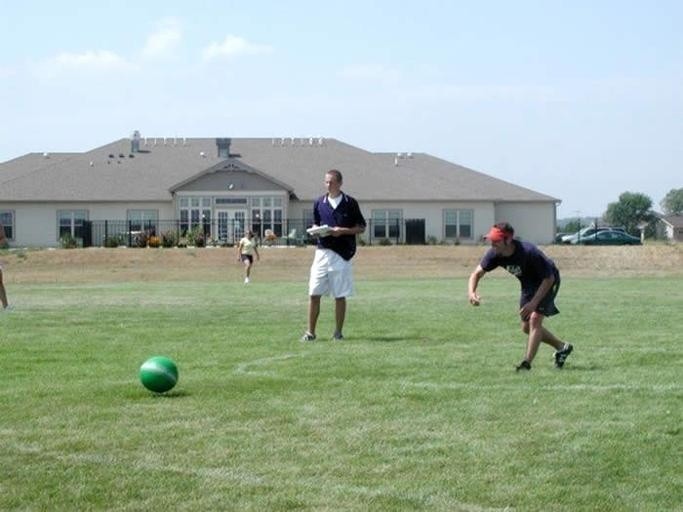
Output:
[469,223,574,371]
[237,231,259,285]
[1,267,13,310]
[301,170,366,340]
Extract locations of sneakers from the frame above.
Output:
[553,342,573,369]
[512,359,531,371]
[299,331,344,341]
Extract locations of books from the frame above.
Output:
[306,224,333,237]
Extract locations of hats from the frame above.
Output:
[482,228,513,241]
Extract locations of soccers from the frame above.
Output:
[140,355,179,393]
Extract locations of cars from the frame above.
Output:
[556,227,641,245]
